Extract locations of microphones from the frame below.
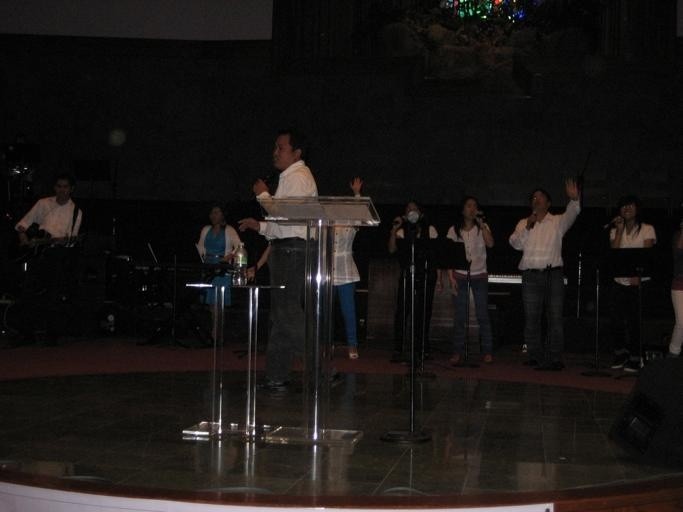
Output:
[256,170,277,192]
[472,212,488,220]
[531,208,537,229]
[391,216,408,224]
[603,213,628,231]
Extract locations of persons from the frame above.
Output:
[197,204,245,346]
[608,197,657,372]
[238,130,320,384]
[14,173,84,249]
[509,178,581,363]
[446,196,494,368]
[669,221,683,355]
[388,200,443,360]
[326,175,362,360]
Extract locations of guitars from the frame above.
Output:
[8,229,78,264]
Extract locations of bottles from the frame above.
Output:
[232,242,247,286]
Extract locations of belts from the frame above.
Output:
[529,266,561,272]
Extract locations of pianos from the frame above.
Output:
[487,273,568,285]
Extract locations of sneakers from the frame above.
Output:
[624,359,644,372]
[453,353,466,365]
[480,353,494,363]
[610,355,629,368]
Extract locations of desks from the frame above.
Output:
[182,284,287,443]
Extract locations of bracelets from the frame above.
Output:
[480,225,487,230]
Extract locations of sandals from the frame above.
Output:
[349,349,359,359]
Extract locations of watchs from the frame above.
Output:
[244,258,264,281]
[570,196,580,201]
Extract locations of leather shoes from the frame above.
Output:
[269,237,303,244]
[243,378,290,392]
[548,360,566,371]
[205,254,224,257]
[526,359,546,368]
[330,369,345,388]
